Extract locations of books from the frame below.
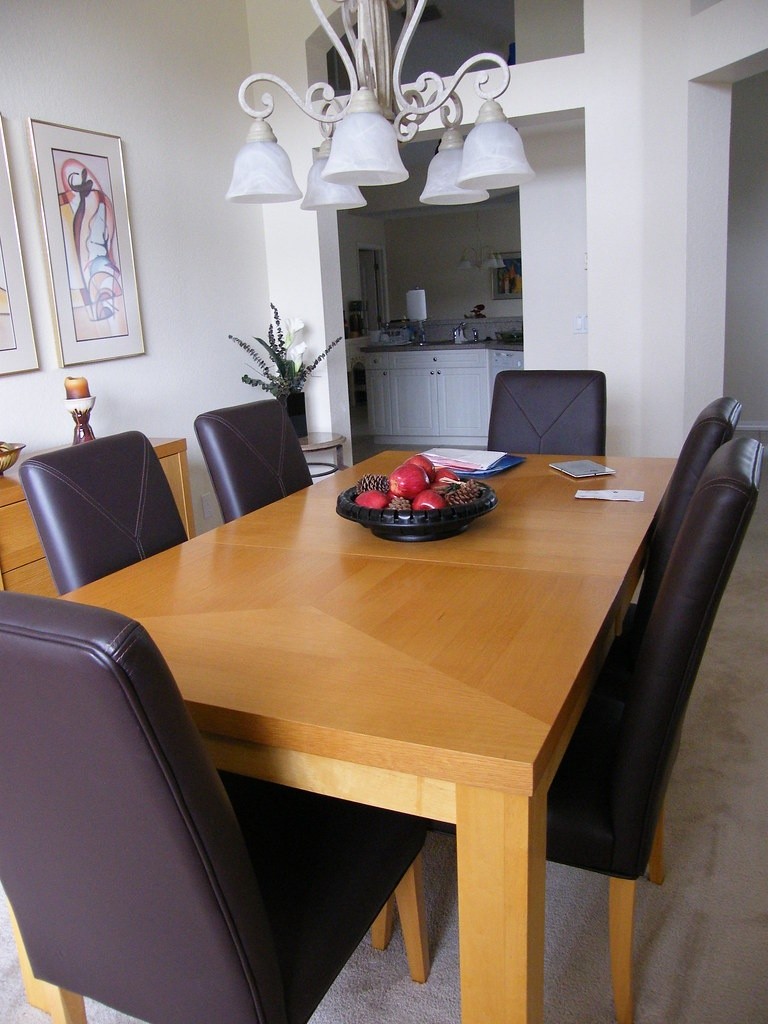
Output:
[423,446,507,472]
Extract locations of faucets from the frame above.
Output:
[452,321,469,344]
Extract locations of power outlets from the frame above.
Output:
[201,491,214,520]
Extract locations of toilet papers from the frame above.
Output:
[406,289,427,321]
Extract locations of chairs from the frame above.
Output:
[0,589,426,1024]
[607,398,742,669]
[18,430,189,595]
[487,370,606,456]
[193,399,313,524]
[371,436,765,1024]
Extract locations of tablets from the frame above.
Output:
[549,459,616,479]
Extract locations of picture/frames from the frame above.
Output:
[490,251,522,300]
[0,111,41,377]
[28,117,147,368]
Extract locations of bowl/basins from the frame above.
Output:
[335,477,498,542]
[0,443,26,478]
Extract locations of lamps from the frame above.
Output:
[456,203,505,274]
[225,0,536,211]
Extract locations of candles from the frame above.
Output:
[64,376,92,399]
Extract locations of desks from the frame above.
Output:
[298,432,346,478]
[6,451,680,1024]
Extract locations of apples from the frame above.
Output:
[388,455,458,510]
[354,491,390,509]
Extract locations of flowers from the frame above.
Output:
[228,301,343,411]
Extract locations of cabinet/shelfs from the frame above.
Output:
[0,438,196,598]
[365,349,524,447]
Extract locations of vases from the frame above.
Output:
[277,392,310,438]
